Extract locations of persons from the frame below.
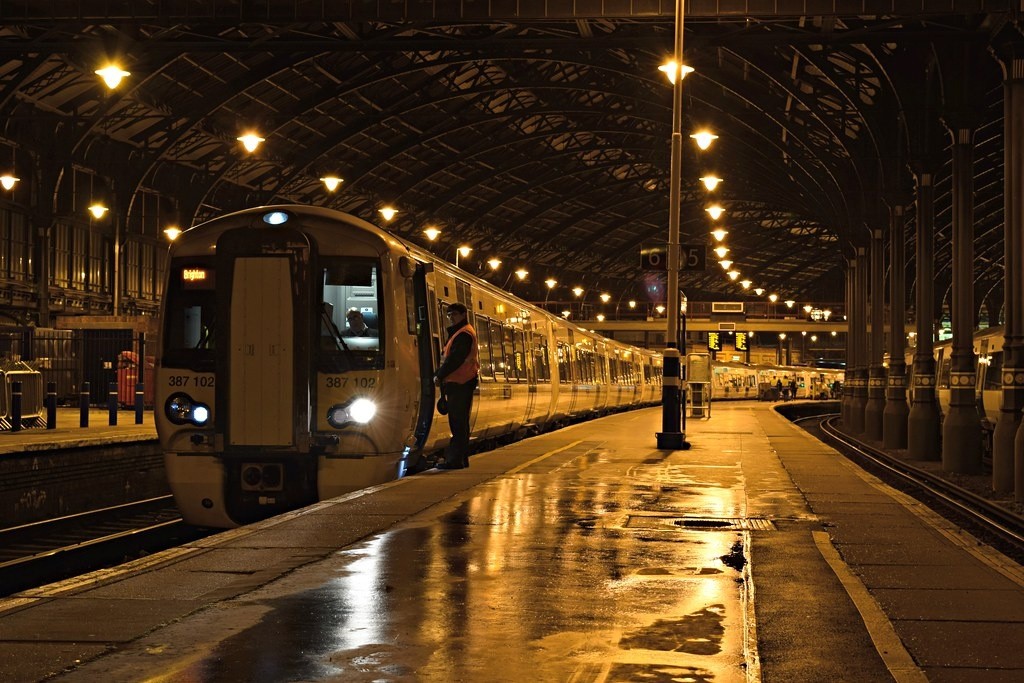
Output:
[433,303,478,468]
[766,369,842,402]
[339,307,377,337]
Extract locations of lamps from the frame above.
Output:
[544,277,666,321]
[754,287,832,316]
[378,206,399,220]
[87,204,109,218]
[710,229,728,241]
[163,227,183,240]
[726,270,740,280]
[320,175,345,191]
[514,268,529,279]
[699,175,724,191]
[237,133,266,152]
[739,279,752,289]
[718,260,733,270]
[690,132,719,150]
[423,227,442,240]
[0,171,20,192]
[705,204,726,220]
[713,247,731,258]
[658,61,695,85]
[459,244,474,256]
[94,65,131,89]
[487,258,503,269]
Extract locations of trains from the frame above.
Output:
[154,204,843,532]
[881,325,1008,450]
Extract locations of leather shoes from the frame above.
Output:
[437,456,469,468]
